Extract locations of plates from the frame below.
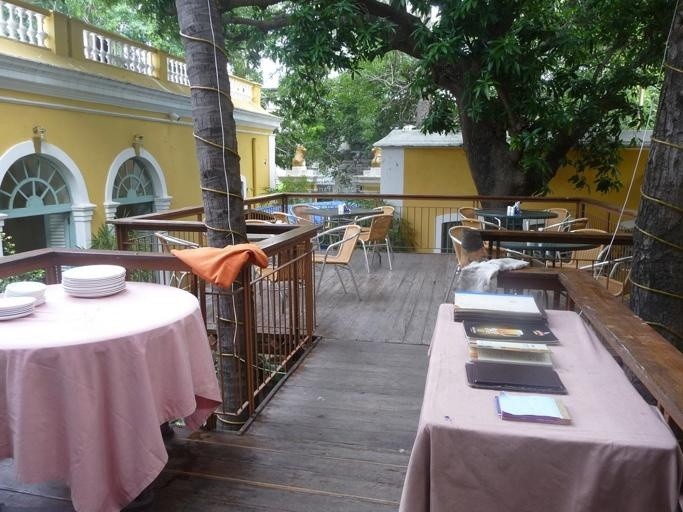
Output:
[0,264,128,323]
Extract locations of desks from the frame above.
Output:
[398,302,683,510]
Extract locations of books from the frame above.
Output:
[451,288,572,426]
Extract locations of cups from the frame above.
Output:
[338,204,344,216]
[507,205,515,216]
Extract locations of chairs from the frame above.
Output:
[443,207,633,305]
[154,233,220,320]
[243,204,394,313]
